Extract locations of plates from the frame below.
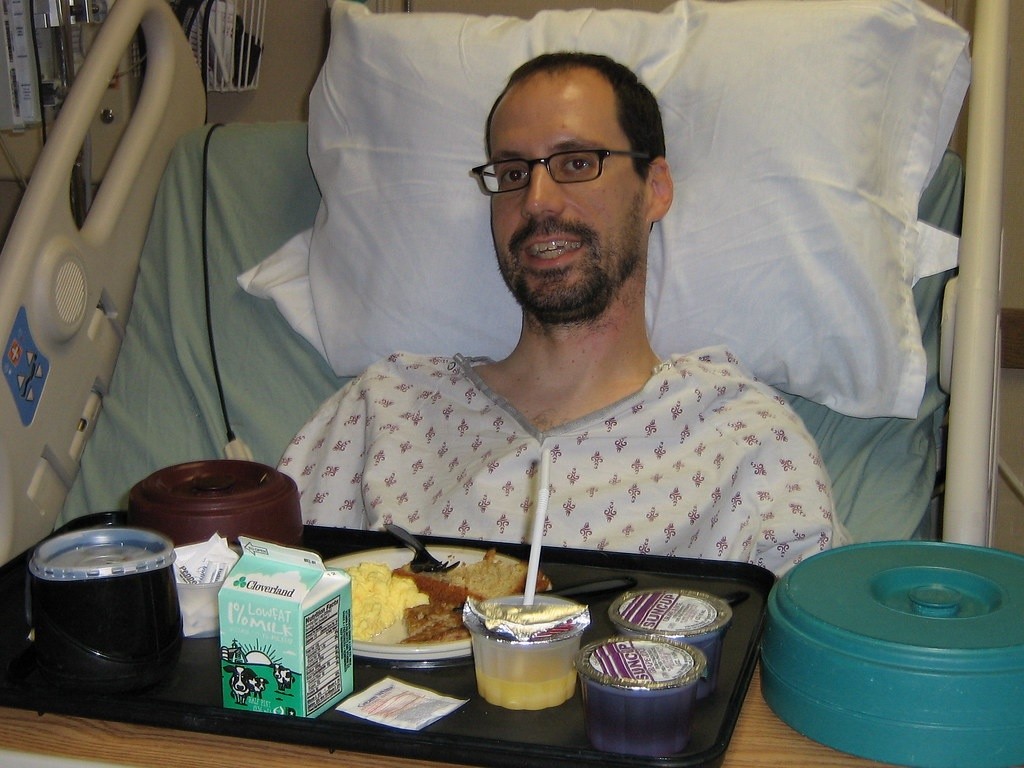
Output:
[322,548,551,659]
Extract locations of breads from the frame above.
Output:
[392,549,550,608]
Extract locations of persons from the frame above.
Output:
[274,51,854,576]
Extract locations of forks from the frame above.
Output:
[383,523,460,572]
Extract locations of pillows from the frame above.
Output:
[236,0,971,419]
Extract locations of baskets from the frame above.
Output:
[125,0,267,93]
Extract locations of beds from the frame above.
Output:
[0,0,1009,768]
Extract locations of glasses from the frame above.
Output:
[472,149,650,193]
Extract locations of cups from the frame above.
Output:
[607,588,733,690]
[575,636,707,755]
[29,527,176,695]
[474,595,583,708]
